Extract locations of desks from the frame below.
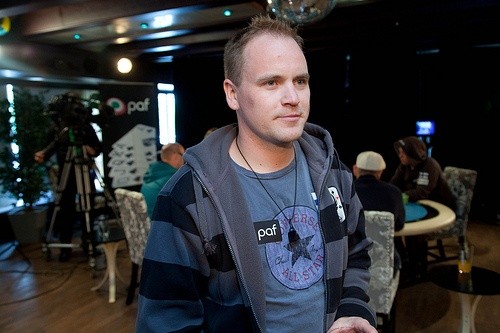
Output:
[393,200,456,282]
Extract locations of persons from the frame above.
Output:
[203,128,222,139]
[34,101,103,261]
[352,151,407,280]
[138,142,187,222]
[389,136,460,281]
[133,13,378,333]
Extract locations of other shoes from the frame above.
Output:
[86,246,102,258]
[58,251,71,262]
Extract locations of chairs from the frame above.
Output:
[115,188,153,306]
[418,166,478,269]
[364,210,400,333]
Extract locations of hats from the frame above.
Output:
[355,151,386,170]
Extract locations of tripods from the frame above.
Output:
[40,125,121,278]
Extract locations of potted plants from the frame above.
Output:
[0,86,62,247]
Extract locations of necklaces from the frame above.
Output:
[235,134,300,243]
